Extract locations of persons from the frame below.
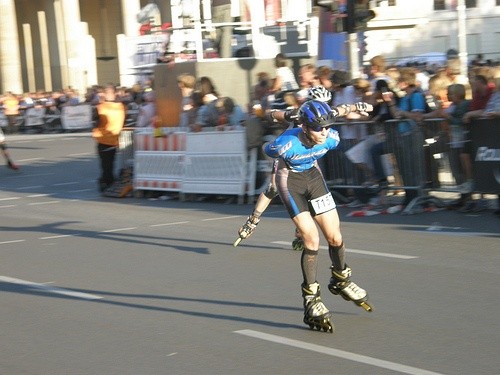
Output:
[265,100,373,333]
[0,48,500,251]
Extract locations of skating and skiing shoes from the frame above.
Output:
[301,281,333,333]
[328,264,372,312]
[232,213,261,247]
[292,228,304,251]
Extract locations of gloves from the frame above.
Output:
[355,101,373,116]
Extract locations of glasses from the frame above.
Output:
[306,125,329,132]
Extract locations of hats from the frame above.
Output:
[332,71,352,84]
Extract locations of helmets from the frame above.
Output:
[298,100,335,127]
[307,86,332,102]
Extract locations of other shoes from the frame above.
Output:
[10,163,18,169]
